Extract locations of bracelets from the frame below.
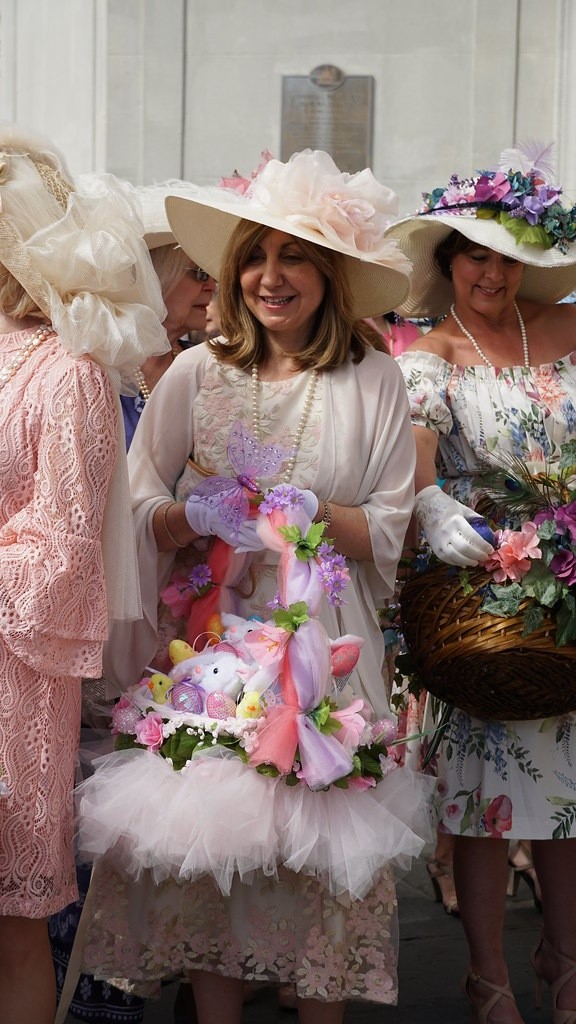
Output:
[163,503,185,548]
[318,499,332,532]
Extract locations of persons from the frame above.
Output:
[357,147,576,1024]
[45,178,223,1024]
[0,141,172,1024]
[65,147,426,1024]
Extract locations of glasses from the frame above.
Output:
[182,265,210,283]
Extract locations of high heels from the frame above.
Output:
[529,925,576,1024]
[426,850,460,918]
[506,841,543,911]
[460,965,525,1024]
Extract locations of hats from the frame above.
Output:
[380,141,576,319]
[164,149,411,319]
[0,125,170,397]
[127,177,197,250]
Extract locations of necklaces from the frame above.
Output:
[0,324,55,390]
[451,301,529,373]
[249,362,317,488]
[137,355,150,398]
[134,368,148,401]
[386,320,394,355]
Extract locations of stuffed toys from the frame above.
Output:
[149,613,363,723]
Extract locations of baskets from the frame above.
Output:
[399,473,576,723]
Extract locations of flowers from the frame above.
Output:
[106,169,576,794]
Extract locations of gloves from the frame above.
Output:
[230,484,319,554]
[185,488,265,546]
[412,485,495,569]
